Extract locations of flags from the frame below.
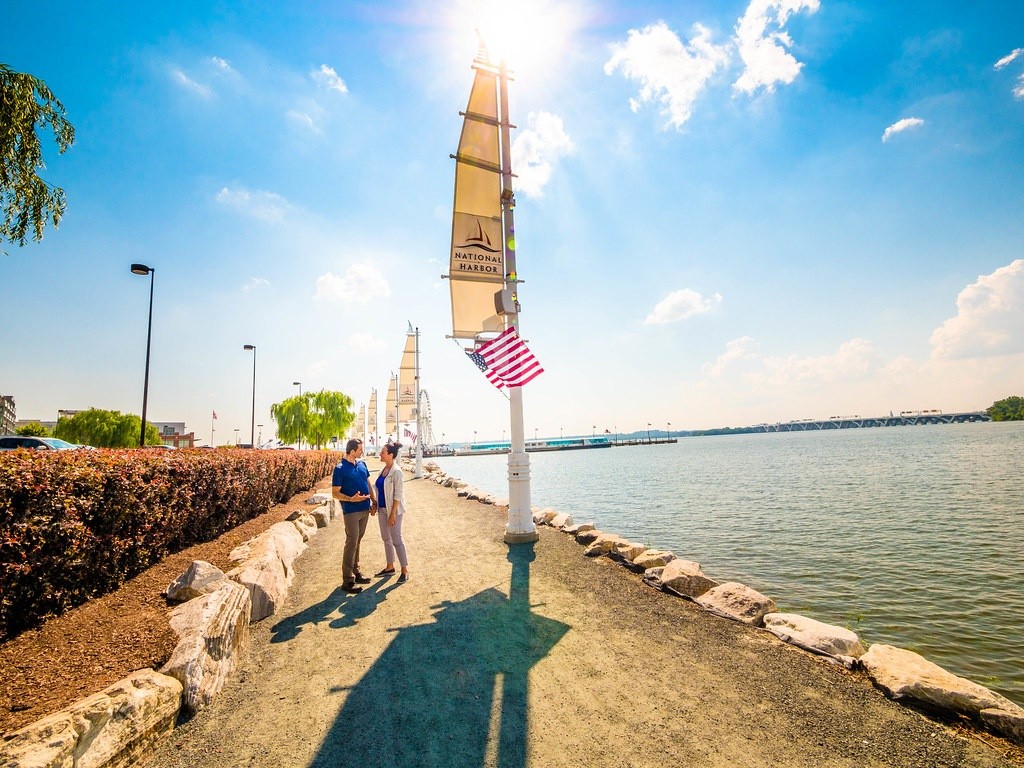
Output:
[213,412,217,419]
[465,325,544,390]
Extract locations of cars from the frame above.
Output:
[236,443,261,450]
[72,444,96,451]
[276,447,295,451]
[195,445,214,450]
[142,445,177,450]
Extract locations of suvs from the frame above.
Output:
[0,435,78,456]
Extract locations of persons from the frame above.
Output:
[332,439,377,593]
[373,442,409,583]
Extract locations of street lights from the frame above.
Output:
[257,424,263,448]
[293,382,301,451]
[243,344,256,450]
[131,262,155,448]
[234,429,240,446]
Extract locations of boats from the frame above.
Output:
[434,433,455,457]
[470,437,613,449]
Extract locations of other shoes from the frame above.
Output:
[342,584,362,594]
[354,576,371,584]
[396,572,410,584]
[375,568,396,577]
